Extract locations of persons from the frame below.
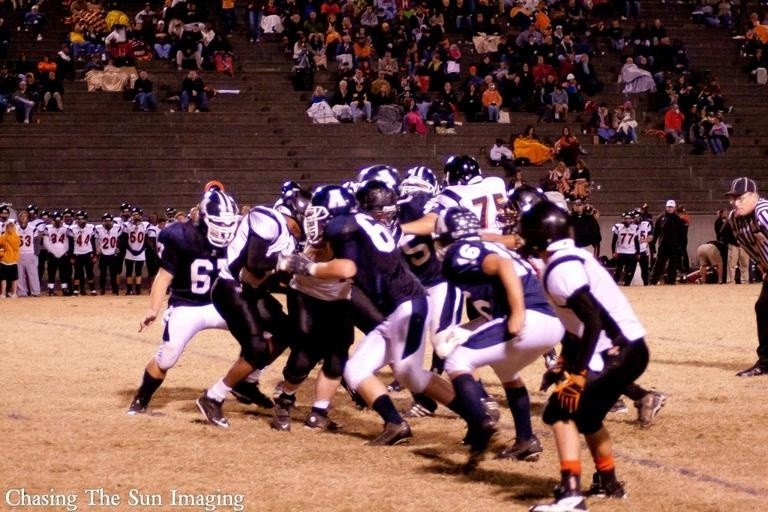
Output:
[1,1,763,205]
[724,175,768,377]
[677,202,693,277]
[646,198,688,286]
[133,154,667,511]
[0,202,201,295]
[698,199,764,283]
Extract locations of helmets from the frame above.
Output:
[77,210,87,218]
[509,185,548,214]
[52,211,63,219]
[360,182,401,231]
[102,212,114,220]
[402,166,439,194]
[621,211,633,218]
[41,210,49,216]
[280,180,300,195]
[27,205,38,212]
[0,205,11,218]
[444,155,482,184]
[274,190,315,242]
[436,208,482,240]
[306,185,354,245]
[167,207,176,214]
[359,163,401,191]
[63,208,74,216]
[120,203,131,211]
[523,203,570,244]
[200,190,240,248]
[633,207,644,218]
[131,207,143,215]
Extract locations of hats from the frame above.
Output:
[725,177,757,196]
[566,73,576,81]
[665,200,676,207]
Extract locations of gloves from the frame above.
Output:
[553,366,591,415]
[287,251,312,277]
[538,363,565,392]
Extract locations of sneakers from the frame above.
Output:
[100,289,105,295]
[480,394,501,421]
[74,288,81,296]
[90,289,98,296]
[306,411,344,431]
[127,288,133,295]
[127,392,151,415]
[405,402,436,418]
[531,494,587,512]
[388,379,406,393]
[273,380,300,410]
[272,403,293,432]
[606,399,629,413]
[62,288,70,296]
[497,438,544,462]
[735,359,768,379]
[134,289,142,295]
[638,388,666,428]
[589,483,628,499]
[46,287,56,296]
[234,383,275,408]
[197,393,229,429]
[464,425,498,474]
[365,421,415,447]
[113,288,120,295]
